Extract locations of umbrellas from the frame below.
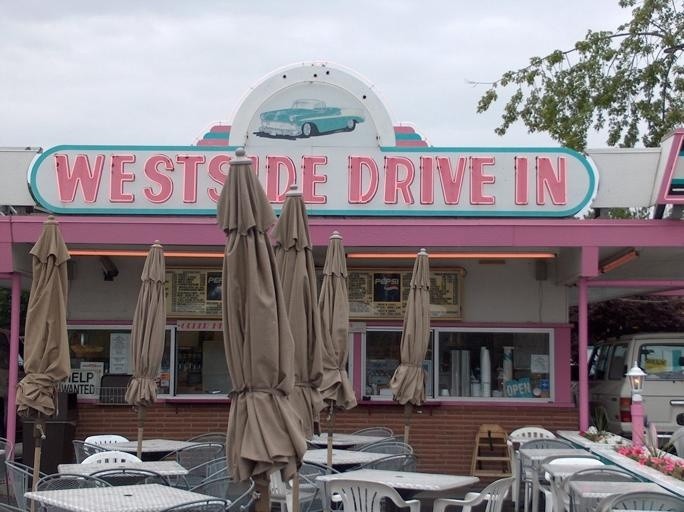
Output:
[15,216,67,512]
[268,183,323,511]
[124,239,177,461]
[389,247,436,450]
[318,228,358,475]
[216,150,310,512]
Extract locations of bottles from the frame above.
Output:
[177,352,200,369]
[470,379,481,396]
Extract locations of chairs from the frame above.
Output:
[599,491,682,512]
[176,456,227,488]
[569,468,643,483]
[324,479,421,511]
[190,476,258,511]
[506,426,557,503]
[530,455,600,511]
[187,432,227,448]
[4,461,53,510]
[356,453,416,470]
[1,437,14,504]
[361,442,414,455]
[81,450,142,463]
[520,439,577,449]
[285,460,343,511]
[33,472,111,510]
[432,476,516,511]
[166,498,227,511]
[160,442,225,487]
[84,434,130,454]
[353,426,393,436]
[73,439,108,463]
[353,437,396,451]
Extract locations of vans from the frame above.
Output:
[0,328,27,436]
[586,332,684,446]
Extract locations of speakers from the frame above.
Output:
[535,259,548,281]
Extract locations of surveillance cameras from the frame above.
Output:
[99,257,120,277]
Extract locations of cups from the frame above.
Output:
[480,345,491,397]
[441,388,449,396]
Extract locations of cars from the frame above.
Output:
[100,348,228,395]
[362,334,550,396]
[255,98,363,138]
[668,425,684,459]
[571,362,581,408]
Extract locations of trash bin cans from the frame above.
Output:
[469,422,513,478]
[21,391,79,486]
